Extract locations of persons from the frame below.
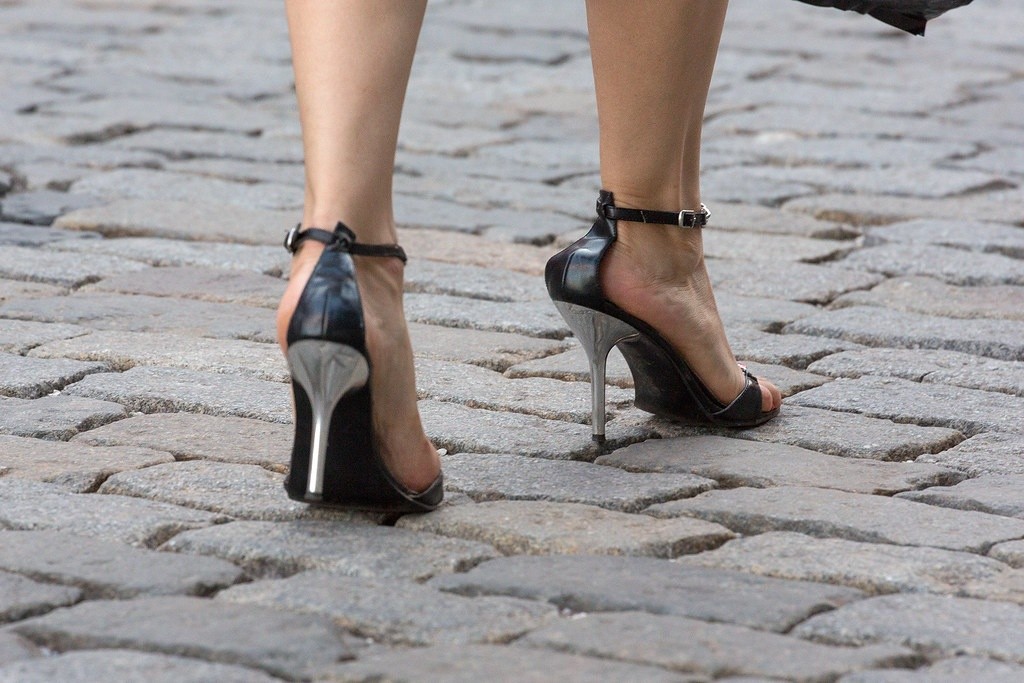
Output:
[274,1,782,515]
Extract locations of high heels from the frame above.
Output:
[545,191,781,442]
[282,222,444,512]
